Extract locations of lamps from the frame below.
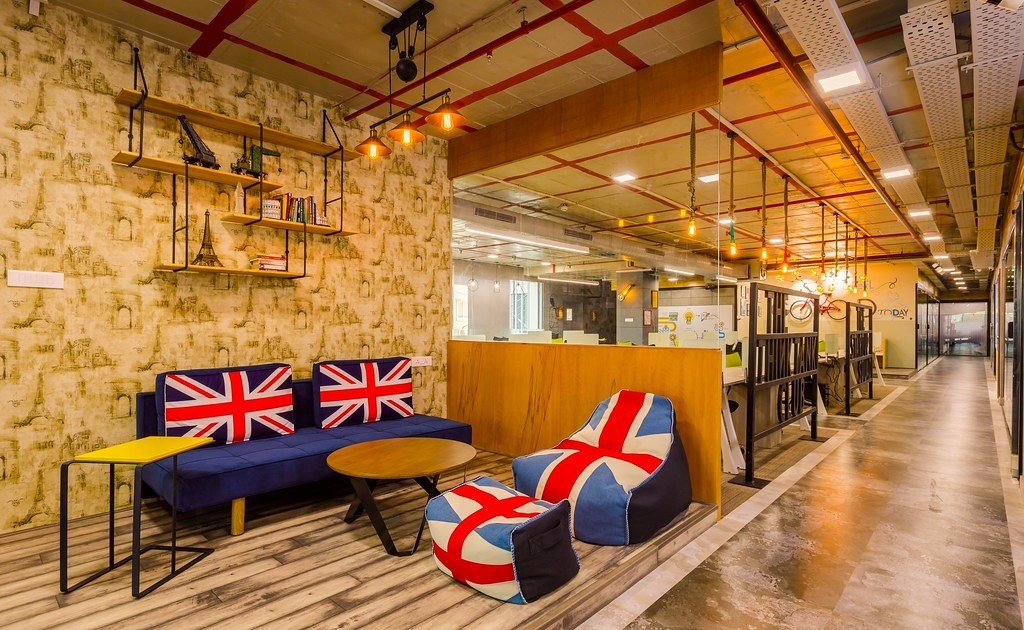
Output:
[386,110,426,146]
[464,223,590,255]
[492,261,500,293]
[819,203,826,280]
[716,274,737,282]
[467,259,479,291]
[354,127,392,158]
[726,131,738,256]
[425,92,468,131]
[829,212,870,296]
[814,62,867,95]
[782,175,791,274]
[759,157,767,258]
[687,112,696,236]
[511,264,528,295]
[663,267,696,276]
[881,165,950,261]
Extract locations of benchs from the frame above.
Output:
[135,378,473,536]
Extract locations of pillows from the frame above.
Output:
[155,363,294,446]
[312,356,414,429]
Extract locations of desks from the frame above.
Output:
[60,436,215,598]
[326,438,476,557]
[817,349,868,407]
[723,365,793,449]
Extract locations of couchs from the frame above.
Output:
[512,388,692,547]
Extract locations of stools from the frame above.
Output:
[423,475,580,605]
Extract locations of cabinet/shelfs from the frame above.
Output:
[111,86,365,279]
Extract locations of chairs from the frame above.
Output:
[873,339,888,369]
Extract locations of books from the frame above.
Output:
[249,253,286,272]
[273,192,318,225]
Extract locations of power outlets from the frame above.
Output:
[411,356,433,367]
